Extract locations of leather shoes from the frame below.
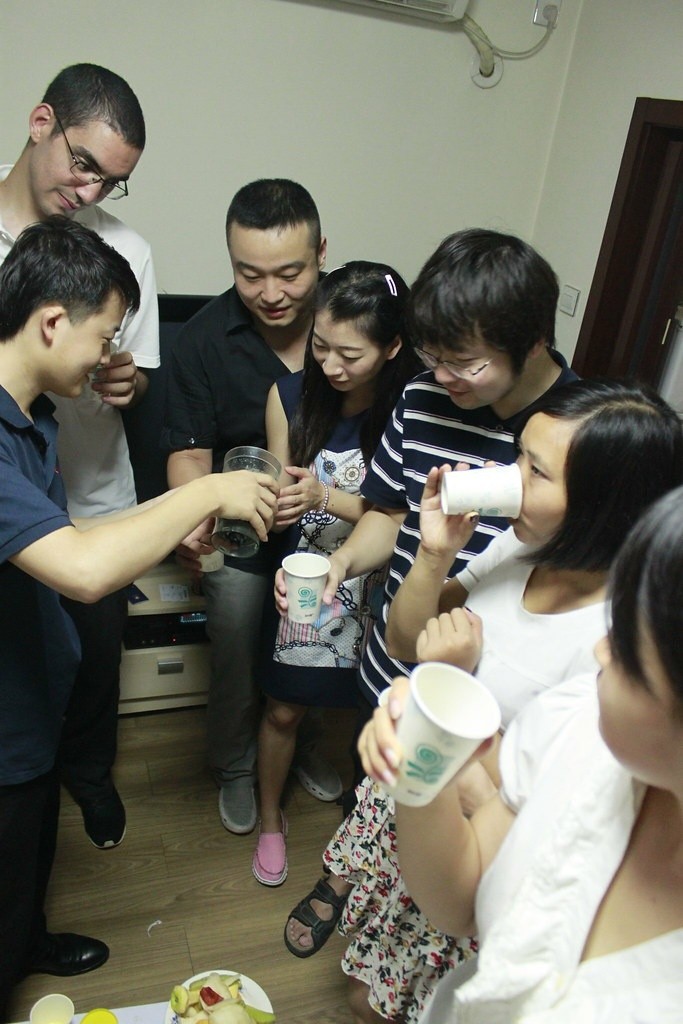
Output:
[24,932,110,977]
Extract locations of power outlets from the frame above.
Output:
[533,0,562,29]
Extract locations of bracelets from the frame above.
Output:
[315,481,329,513]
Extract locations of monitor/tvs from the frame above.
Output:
[118,294,219,506]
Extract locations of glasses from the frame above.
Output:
[413,344,508,382]
[53,111,129,200]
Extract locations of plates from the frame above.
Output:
[79,1008,118,1024]
[165,970,274,1024]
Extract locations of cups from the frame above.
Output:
[193,536,225,572]
[441,463,522,520]
[211,448,278,558]
[281,553,331,623]
[368,661,501,807]
[89,344,119,400]
[29,994,74,1024]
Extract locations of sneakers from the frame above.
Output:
[80,785,127,849]
[218,786,257,834]
[292,751,343,802]
[251,808,289,886]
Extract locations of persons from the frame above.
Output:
[1,214,281,992]
[357,488,683,1024]
[274,229,586,958]
[322,377,683,1024]
[252,259,425,888]
[1,62,160,850]
[165,178,343,833]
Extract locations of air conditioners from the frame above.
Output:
[337,0,468,23]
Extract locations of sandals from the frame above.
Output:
[284,875,351,959]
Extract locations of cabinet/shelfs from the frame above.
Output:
[117,562,211,715]
